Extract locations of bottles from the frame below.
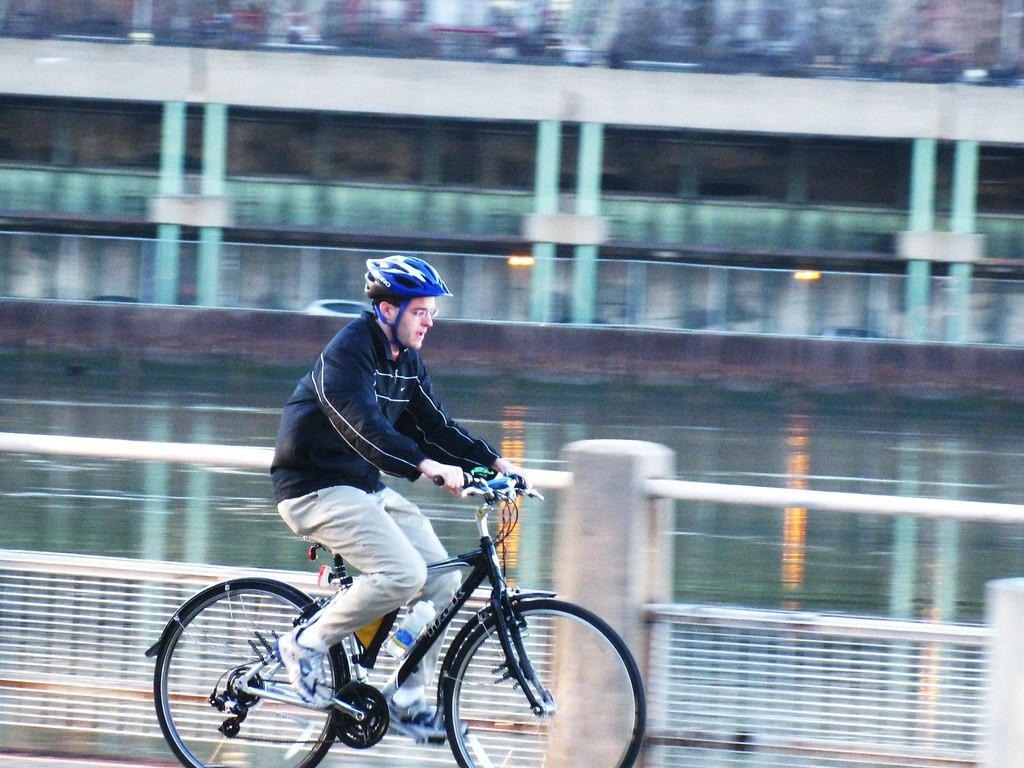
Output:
[382,600,437,659]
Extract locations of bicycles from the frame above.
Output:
[143,467,646,768]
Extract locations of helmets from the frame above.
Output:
[364,255,454,299]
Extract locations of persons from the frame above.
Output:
[269,255,534,740]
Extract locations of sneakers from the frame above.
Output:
[278,626,329,708]
[387,696,467,737]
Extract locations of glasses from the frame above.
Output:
[393,306,438,319]
